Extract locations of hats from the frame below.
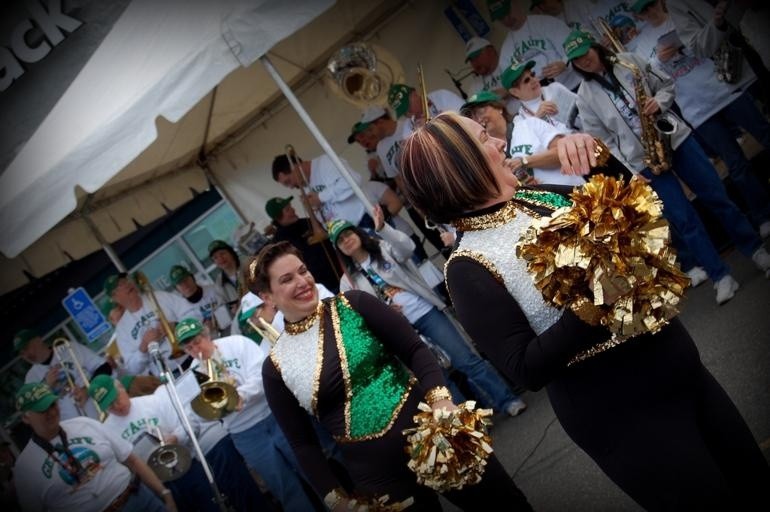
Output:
[12,331,34,352]
[101,273,133,320]
[237,291,264,320]
[348,82,413,144]
[208,239,227,258]
[265,196,294,214]
[626,0,650,14]
[87,375,120,410]
[14,383,60,415]
[169,266,190,286]
[328,218,353,246]
[233,223,255,245]
[462,1,593,113]
[174,319,203,345]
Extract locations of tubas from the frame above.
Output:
[189,350,241,419]
[147,442,191,481]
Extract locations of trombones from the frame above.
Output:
[131,269,184,384]
[54,336,108,425]
[285,144,350,281]
[407,60,441,230]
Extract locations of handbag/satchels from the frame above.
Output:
[416,328,452,370]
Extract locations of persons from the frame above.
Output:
[171,319,324,511]
[117,373,273,511]
[13,0,769,434]
[403,110,768,511]
[14,381,181,511]
[243,241,536,511]
[88,373,224,511]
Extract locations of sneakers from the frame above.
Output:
[684,265,706,287]
[713,274,739,303]
[506,400,525,417]
[753,221,770,269]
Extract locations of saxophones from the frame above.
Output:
[607,56,679,174]
[714,25,745,85]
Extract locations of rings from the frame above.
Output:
[577,144,585,150]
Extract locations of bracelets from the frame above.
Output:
[160,488,172,496]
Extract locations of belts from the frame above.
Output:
[105,481,135,511]
